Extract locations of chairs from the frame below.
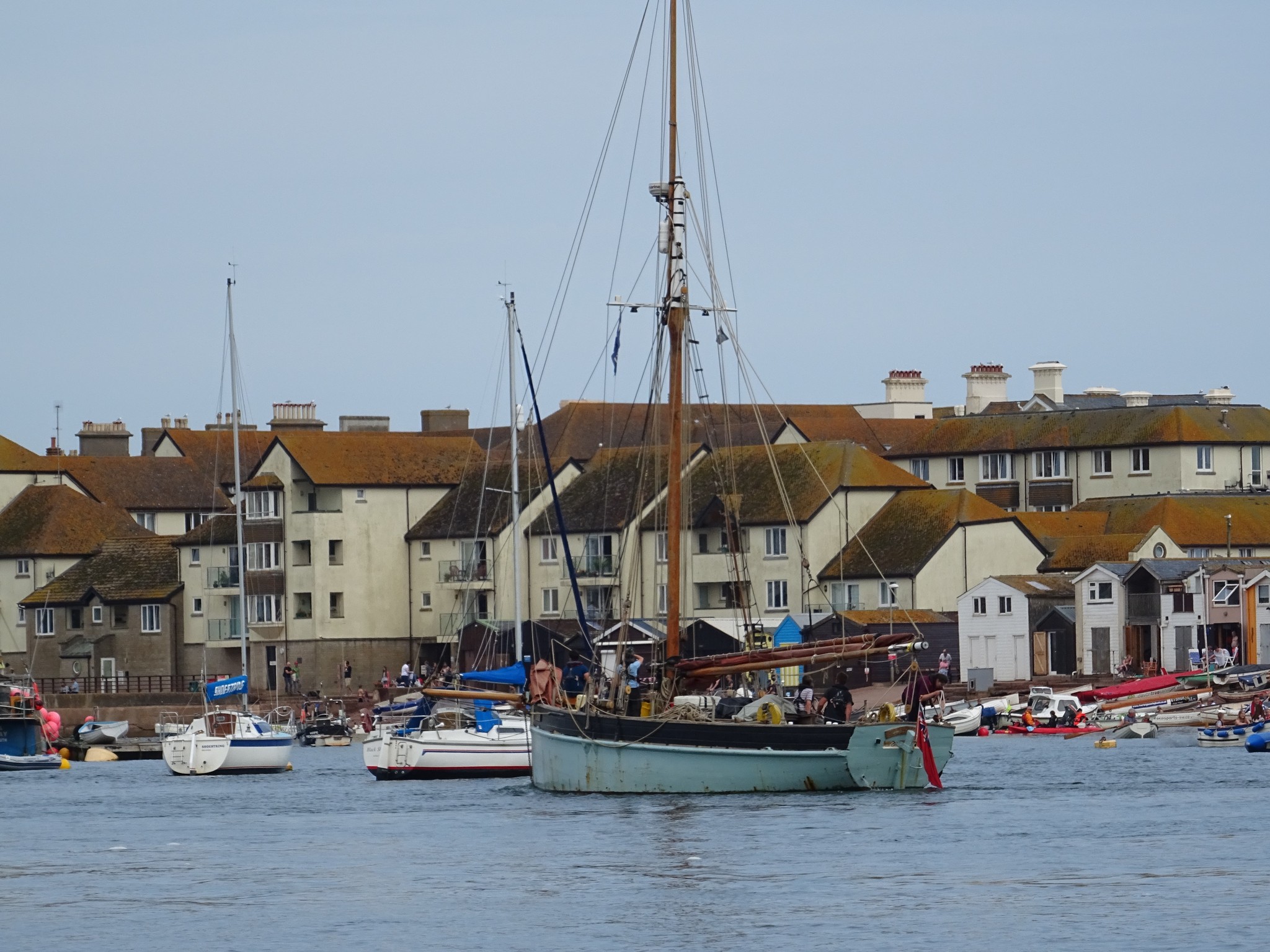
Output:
[449,565,466,582]
[474,564,487,580]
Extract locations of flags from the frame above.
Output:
[917,704,943,789]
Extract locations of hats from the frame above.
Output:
[726,674,731,680]
[294,662,298,664]
[1157,706,1161,709]
[406,661,412,664]
[1128,708,1138,714]
[287,662,291,664]
[71,678,76,680]
[1145,715,1150,719]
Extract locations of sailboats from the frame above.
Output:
[160,276,298,775]
[529,0,962,794]
[1101,574,1213,717]
[361,282,536,781]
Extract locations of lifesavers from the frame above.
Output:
[757,702,782,724]
[877,702,896,722]
[33,683,43,711]
[359,708,373,733]
[300,709,305,724]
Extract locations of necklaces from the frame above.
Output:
[1045,711,1059,728]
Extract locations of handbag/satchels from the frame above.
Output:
[1150,657,1155,667]
[291,673,296,681]
[410,671,414,679]
[383,683,389,688]
[938,668,948,676]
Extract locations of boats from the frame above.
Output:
[921,662,1270,753]
[1112,722,1159,741]
[155,722,192,736]
[78,716,130,745]
[294,682,353,747]
[0,588,66,772]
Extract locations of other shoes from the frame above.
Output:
[344,693,355,697]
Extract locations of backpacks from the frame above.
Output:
[1067,709,1076,719]
[1022,712,1028,723]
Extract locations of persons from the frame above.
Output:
[725,674,734,698]
[817,671,854,725]
[424,661,451,677]
[1208,698,1216,705]
[1232,631,1239,666]
[381,666,390,688]
[283,662,293,693]
[1155,706,1164,713]
[344,661,354,694]
[1123,708,1150,725]
[357,684,366,703]
[291,662,302,693]
[793,675,814,714]
[1203,645,1224,669]
[67,678,79,694]
[400,661,413,682]
[1196,697,1205,708]
[939,649,952,684]
[59,680,70,694]
[1021,706,1040,727]
[563,649,592,707]
[902,674,948,721]
[616,647,644,716]
[706,679,722,696]
[1215,694,1270,728]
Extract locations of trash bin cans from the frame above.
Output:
[189,681,198,692]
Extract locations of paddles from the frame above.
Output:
[1064,725,1122,740]
